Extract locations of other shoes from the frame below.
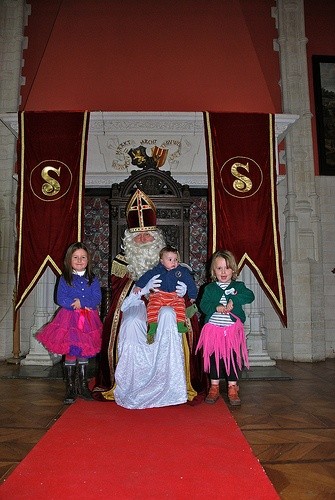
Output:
[227,384,241,406]
[204,384,219,404]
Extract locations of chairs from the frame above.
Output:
[102,156,191,321]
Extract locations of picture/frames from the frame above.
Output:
[312,54,335,176]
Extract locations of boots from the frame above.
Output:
[75,361,94,400]
[64,364,76,404]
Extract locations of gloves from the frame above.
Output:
[141,273,162,296]
[175,281,187,298]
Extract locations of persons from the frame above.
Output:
[199,250,256,407]
[102,187,208,411]
[36,242,105,406]
[132,246,198,345]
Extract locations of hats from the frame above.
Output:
[126,189,157,232]
